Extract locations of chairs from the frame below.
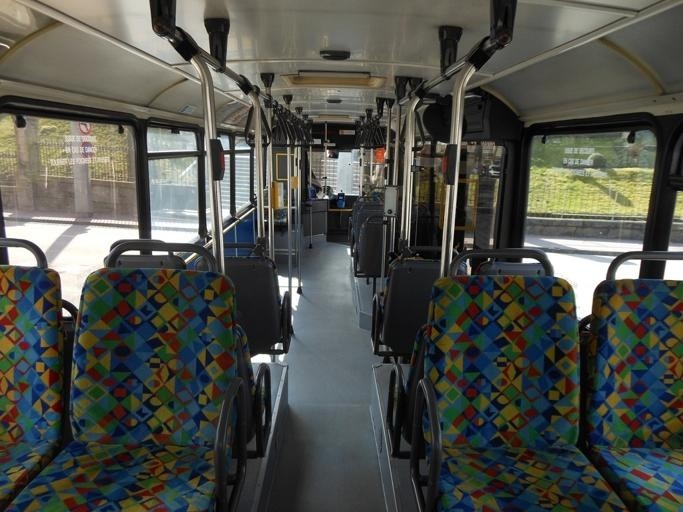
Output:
[351,215,384,277]
[351,204,384,257]
[349,196,383,240]
[103,240,272,454]
[1,240,247,512]
[194,243,292,353]
[583,252,683,512]
[410,247,628,512]
[1,238,73,512]
[371,247,467,355]
[387,246,550,457]
